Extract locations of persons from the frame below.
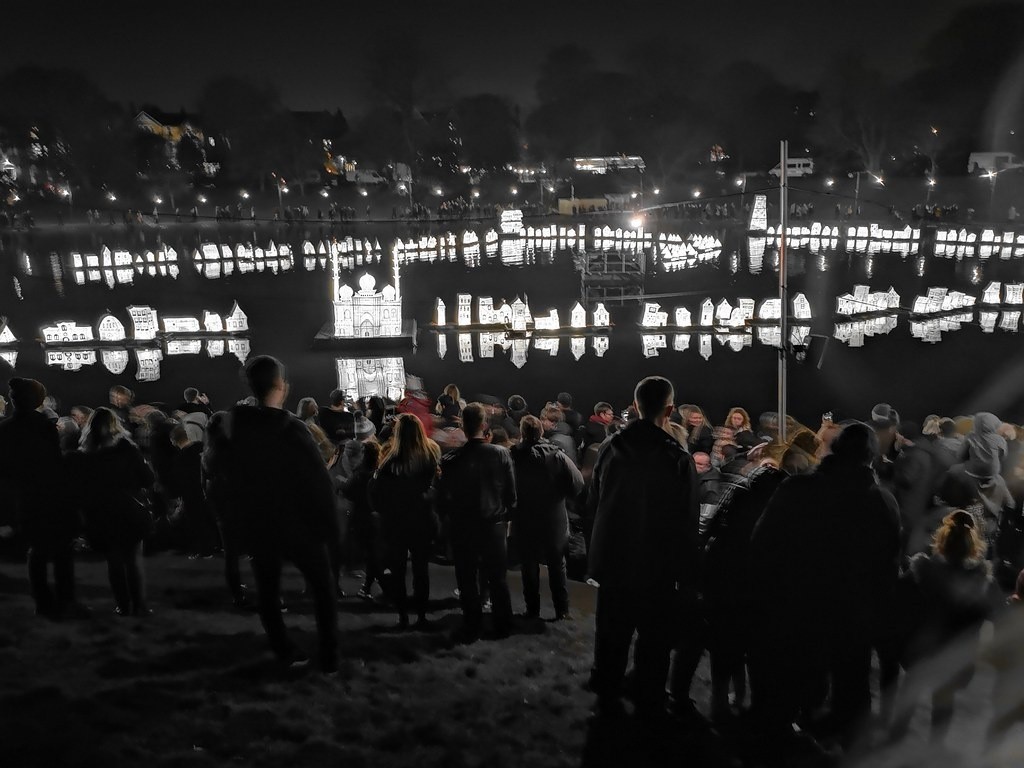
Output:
[0,197,1019,230]
[0,355,1024,768]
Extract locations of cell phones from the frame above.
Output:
[825,413,832,420]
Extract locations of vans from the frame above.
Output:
[346,169,383,182]
[968,152,1023,174]
[769,158,814,179]
